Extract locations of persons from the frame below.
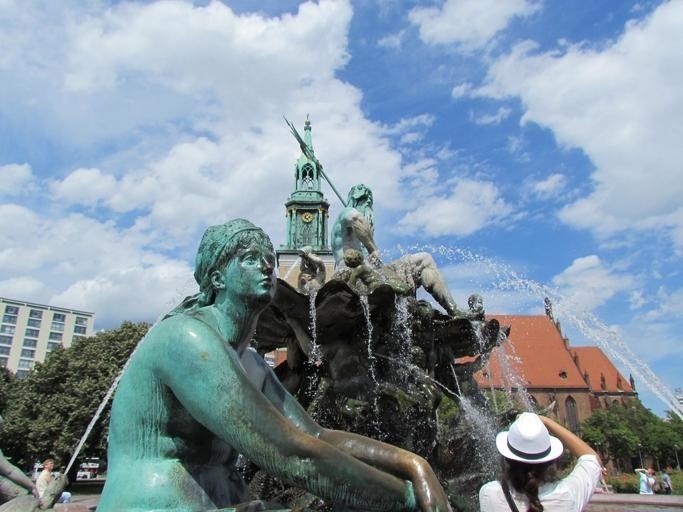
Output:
[633,467,656,495]
[478,411,602,512]
[34,458,72,504]
[343,246,384,295]
[328,182,488,321]
[598,462,613,495]
[651,471,659,494]
[466,292,513,340]
[84,217,456,512]
[661,468,671,495]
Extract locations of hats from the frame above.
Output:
[494,411,566,466]
